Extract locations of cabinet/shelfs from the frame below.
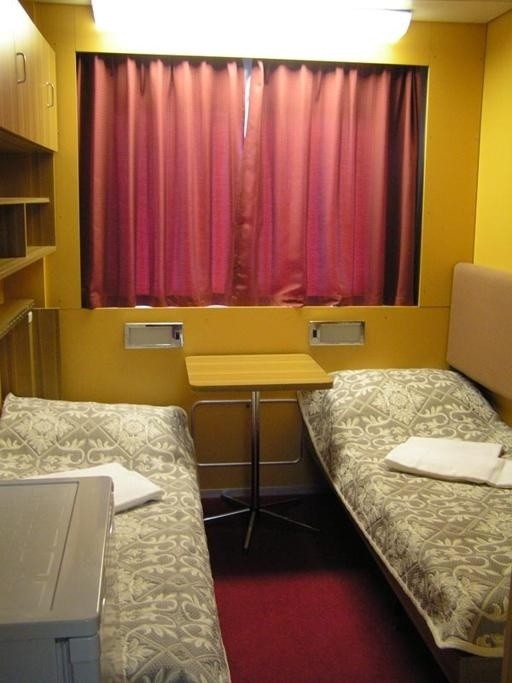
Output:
[1,128,56,336]
[35,20,58,154]
[0,0,35,141]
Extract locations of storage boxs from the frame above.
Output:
[0,475,115,664]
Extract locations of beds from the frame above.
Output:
[0,393,231,682]
[298,368,512,681]
